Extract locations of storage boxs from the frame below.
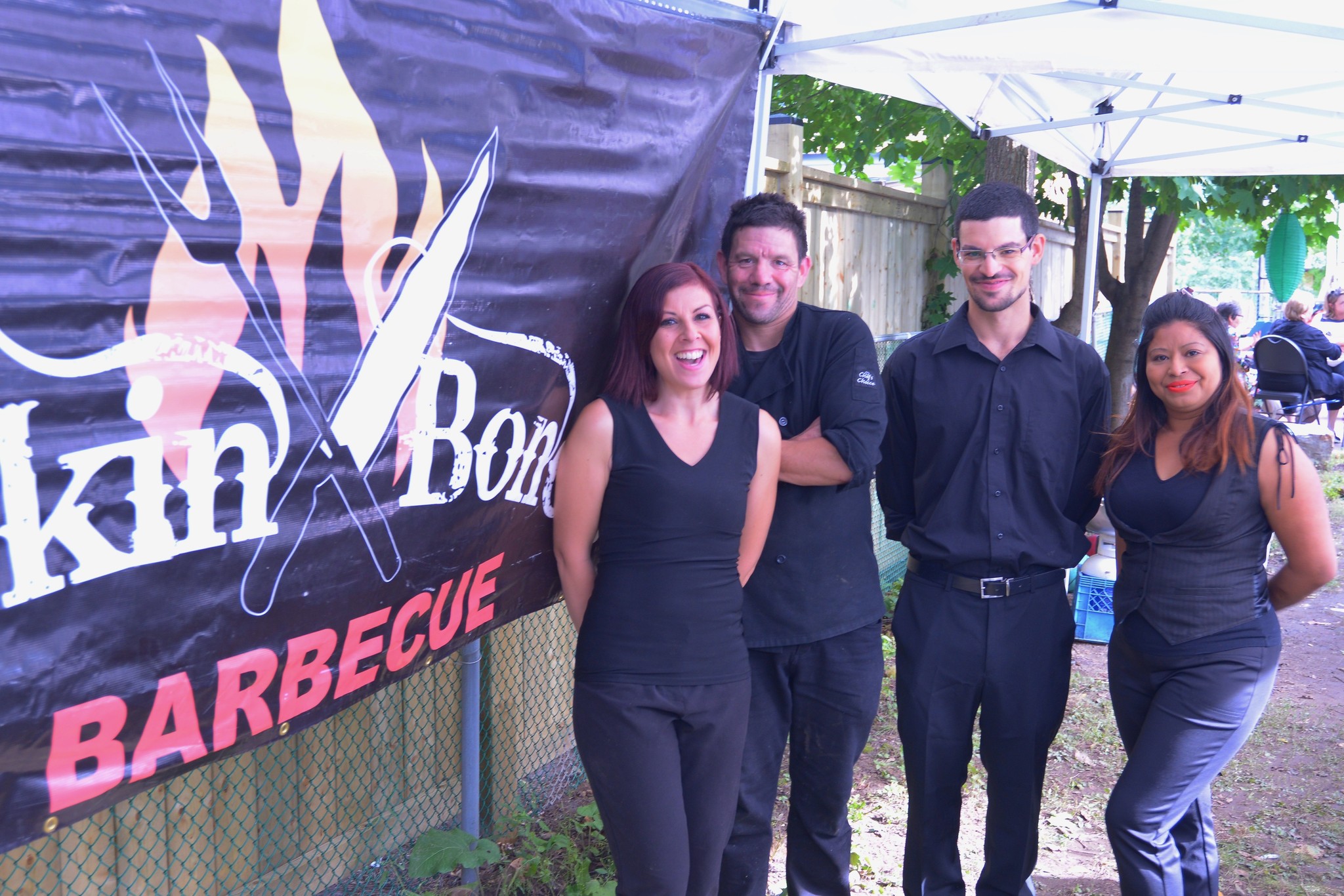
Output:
[1071,564,1115,643]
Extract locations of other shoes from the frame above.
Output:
[1334,433,1340,443]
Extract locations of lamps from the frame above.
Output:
[1265,208,1306,303]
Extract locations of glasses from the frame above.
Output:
[956,236,1036,262]
[1236,314,1244,319]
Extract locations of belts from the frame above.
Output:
[906,554,1066,600]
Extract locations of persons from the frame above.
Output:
[1091,292,1338,896]
[1215,286,1344,444]
[872,185,1113,896]
[718,192,889,896]
[552,261,781,895]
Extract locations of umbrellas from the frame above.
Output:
[717,0,1344,344]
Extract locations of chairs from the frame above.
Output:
[1254,334,1325,425]
